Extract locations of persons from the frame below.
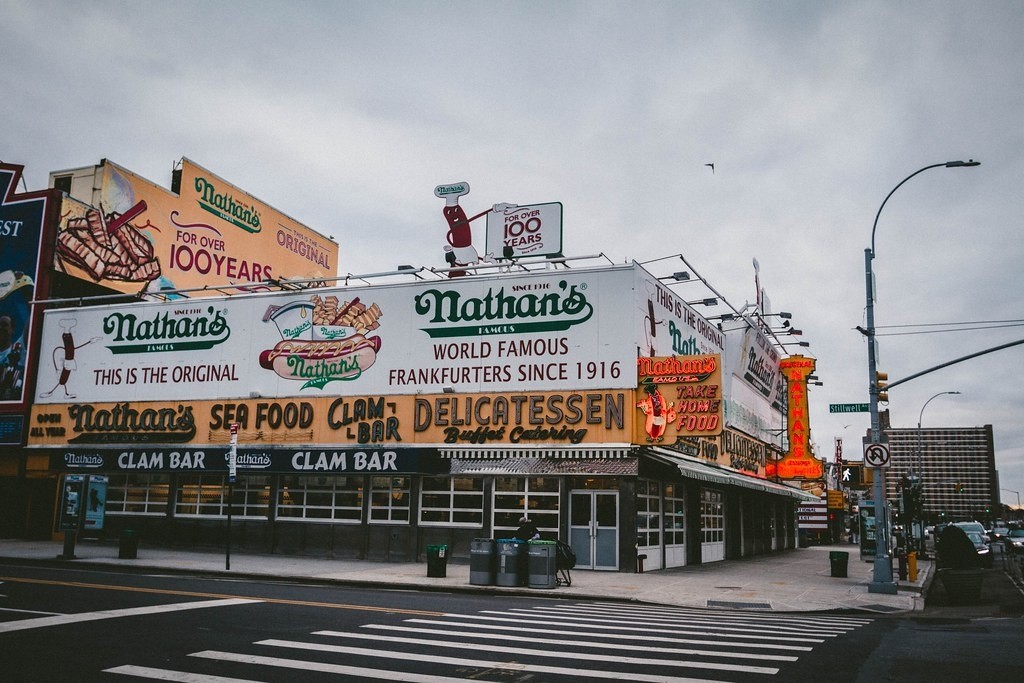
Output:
[512,517,541,541]
[0,315,32,365]
[853,524,859,543]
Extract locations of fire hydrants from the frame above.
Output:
[898,553,908,580]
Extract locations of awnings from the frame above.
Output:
[650,452,730,484]
[716,468,765,492]
[752,478,791,497]
[777,484,821,502]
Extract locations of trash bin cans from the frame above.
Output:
[468,537,495,585]
[424,543,448,577]
[829,551,849,577]
[495,539,526,587]
[118,529,138,559]
[526,540,558,588]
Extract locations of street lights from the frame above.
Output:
[869,160,981,594]
[919,391,962,560]
[1002,489,1020,509]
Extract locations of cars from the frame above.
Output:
[925,527,934,533]
[991,519,1023,526]
[934,525,947,545]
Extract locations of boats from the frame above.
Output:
[1006,529,1024,550]
[993,528,1012,541]
[963,531,994,568]
[947,520,991,543]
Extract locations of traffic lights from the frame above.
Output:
[985,506,990,513]
[957,483,963,492]
[876,371,887,401]
